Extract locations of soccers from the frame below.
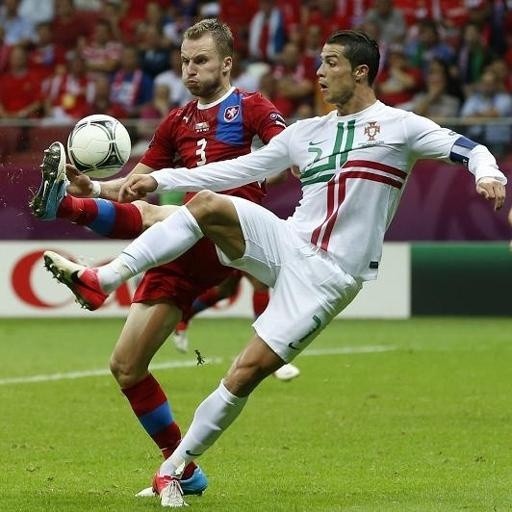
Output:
[68,113,130,178]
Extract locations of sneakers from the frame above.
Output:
[29,142,70,222]
[43,250,109,311]
[136,465,209,496]
[151,470,188,507]
[168,330,188,353]
[274,364,301,382]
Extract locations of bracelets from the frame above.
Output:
[88,178,101,199]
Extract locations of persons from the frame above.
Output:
[29,17,285,496]
[42,28,507,507]
